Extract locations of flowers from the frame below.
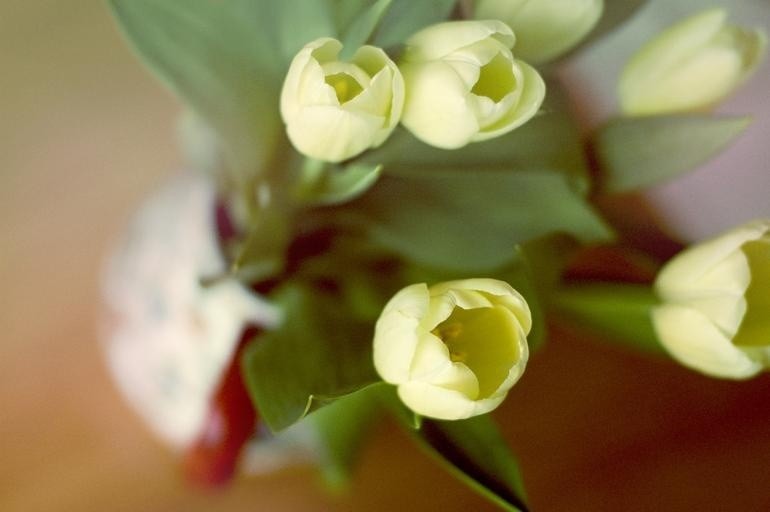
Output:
[101,1,770,512]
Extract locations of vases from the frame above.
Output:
[102,164,322,479]
